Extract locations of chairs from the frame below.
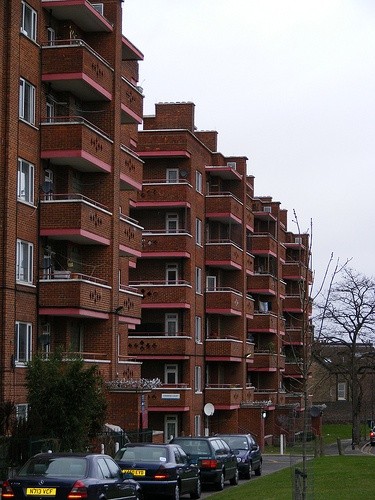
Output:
[199,445,208,451]
[126,452,135,459]
[34,464,47,474]
[70,464,82,473]
[151,452,163,459]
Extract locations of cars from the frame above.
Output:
[2,451,144,500]
[370,426,375,447]
[114,442,202,500]
[212,433,262,480]
[169,437,239,490]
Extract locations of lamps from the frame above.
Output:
[262,410,266,419]
